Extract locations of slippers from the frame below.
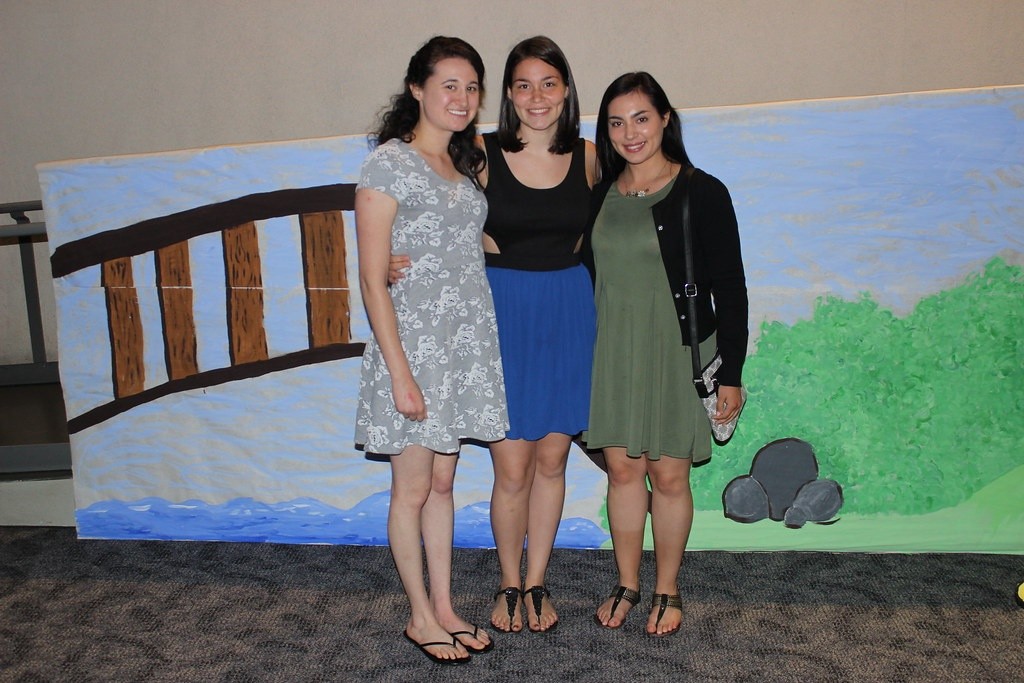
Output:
[489,586,524,635]
[450,621,494,654]
[521,585,560,635]
[402,627,473,665]
[646,593,683,639]
[593,584,641,631]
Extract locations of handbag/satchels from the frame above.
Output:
[692,352,748,446]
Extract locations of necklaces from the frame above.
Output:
[626,159,667,198]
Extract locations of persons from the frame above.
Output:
[383,34,602,633]
[581,69,748,637]
[354,34,511,665]
